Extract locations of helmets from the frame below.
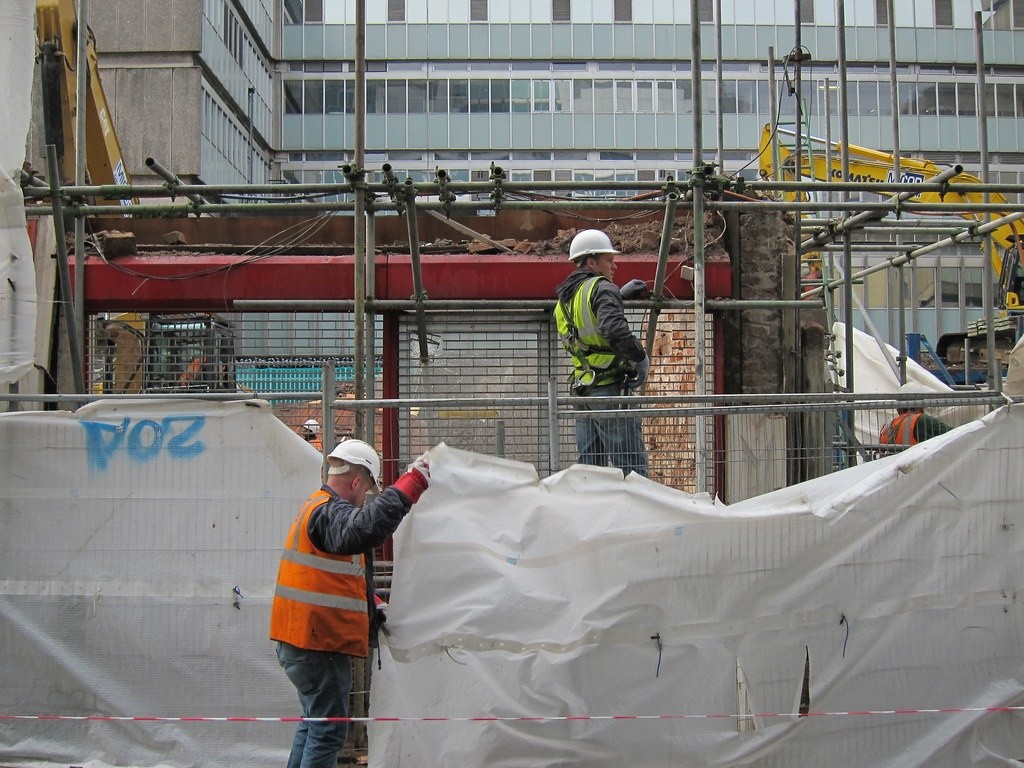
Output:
[896,382,926,394]
[568,228,621,260]
[328,438,380,487]
[304,419,320,435]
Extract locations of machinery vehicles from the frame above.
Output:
[29,0,240,410]
[755,121,1024,367]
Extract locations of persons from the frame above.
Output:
[302,419,323,454]
[879,382,954,458]
[271,438,429,768]
[552,228,651,480]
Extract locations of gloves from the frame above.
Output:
[394,456,431,503]
[619,279,648,299]
[628,354,650,388]
